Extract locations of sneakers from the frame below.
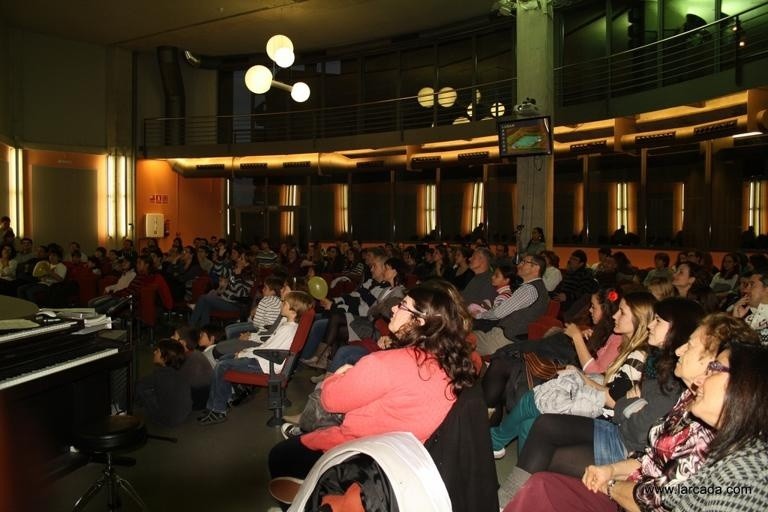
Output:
[226,387,254,407]
[310,374,325,384]
[493,448,506,459]
[269,476,305,505]
[281,423,302,440]
[198,409,226,425]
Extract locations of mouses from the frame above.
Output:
[36,311,57,319]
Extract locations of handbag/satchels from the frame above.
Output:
[524,351,565,379]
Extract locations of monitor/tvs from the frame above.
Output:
[497,116,552,158]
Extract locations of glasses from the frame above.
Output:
[398,301,423,317]
[521,260,538,267]
[708,361,730,372]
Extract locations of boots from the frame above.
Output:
[498,466,532,512]
[299,343,327,367]
[315,347,331,369]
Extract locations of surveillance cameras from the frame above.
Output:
[514,102,539,114]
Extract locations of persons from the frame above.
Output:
[1,212,767,511]
